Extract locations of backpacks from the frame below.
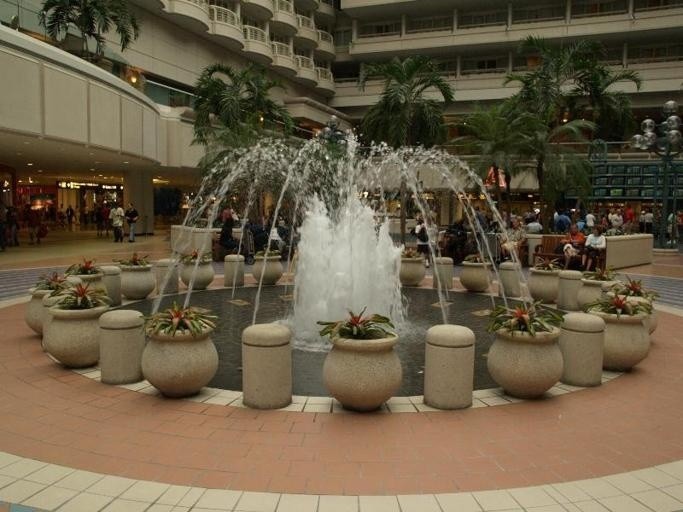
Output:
[416,227,427,242]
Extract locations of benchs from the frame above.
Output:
[435,233,482,262]
[532,236,605,276]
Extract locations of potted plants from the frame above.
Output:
[459,248,494,292]
[576,263,659,373]
[139,300,223,399]
[111,252,155,300]
[24,254,115,369]
[176,247,215,290]
[528,257,565,304]
[316,305,403,413]
[252,246,284,285]
[399,248,426,286]
[486,297,564,400]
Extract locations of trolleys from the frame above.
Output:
[240,219,256,266]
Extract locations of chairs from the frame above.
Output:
[497,235,529,268]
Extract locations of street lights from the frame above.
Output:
[631,98,682,248]
[313,113,351,151]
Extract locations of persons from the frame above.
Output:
[219,206,243,255]
[413,202,683,271]
[0,199,139,252]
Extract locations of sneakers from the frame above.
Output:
[9,242,14,246]
[29,242,34,246]
[114,239,118,243]
[1,248,5,252]
[128,240,135,242]
[15,243,19,246]
[36,240,40,243]
[120,238,123,242]
